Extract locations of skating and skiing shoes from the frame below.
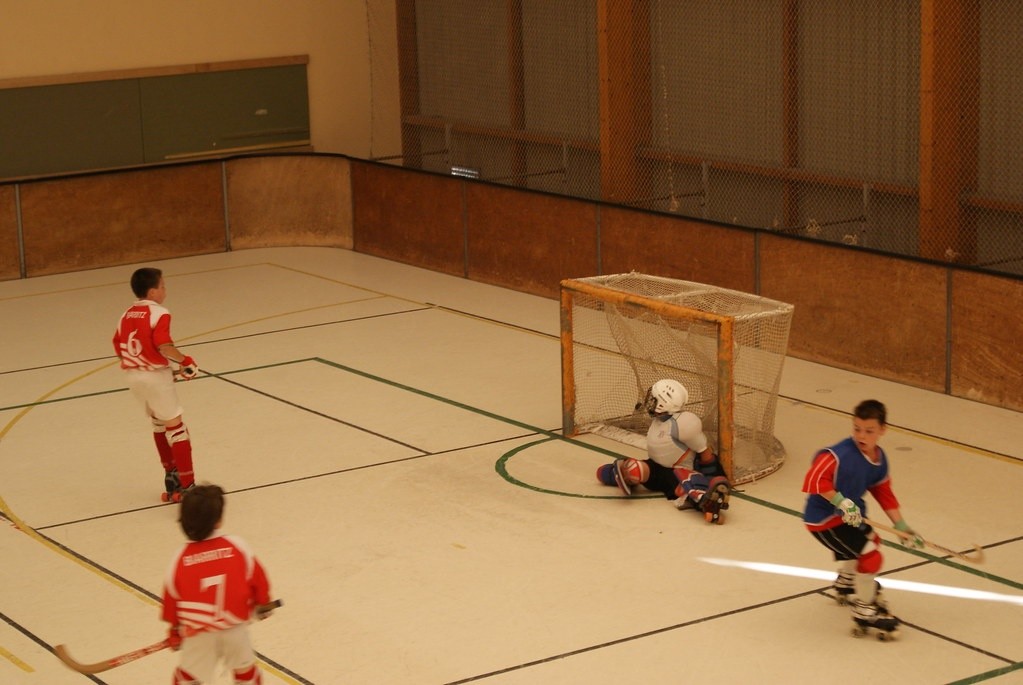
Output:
[597,463,616,484]
[698,476,730,525]
[851,598,898,640]
[162,467,182,502]
[833,571,880,606]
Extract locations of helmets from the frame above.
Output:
[644,379,688,422]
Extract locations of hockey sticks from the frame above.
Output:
[862,518,985,564]
[53,598,284,673]
[173,368,193,375]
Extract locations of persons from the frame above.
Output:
[800,399,926,641]
[160,484,270,685]
[113,268,198,501]
[597,379,730,525]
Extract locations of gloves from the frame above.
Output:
[179,355,198,381]
[894,519,925,550]
[170,627,183,650]
[830,491,862,528]
